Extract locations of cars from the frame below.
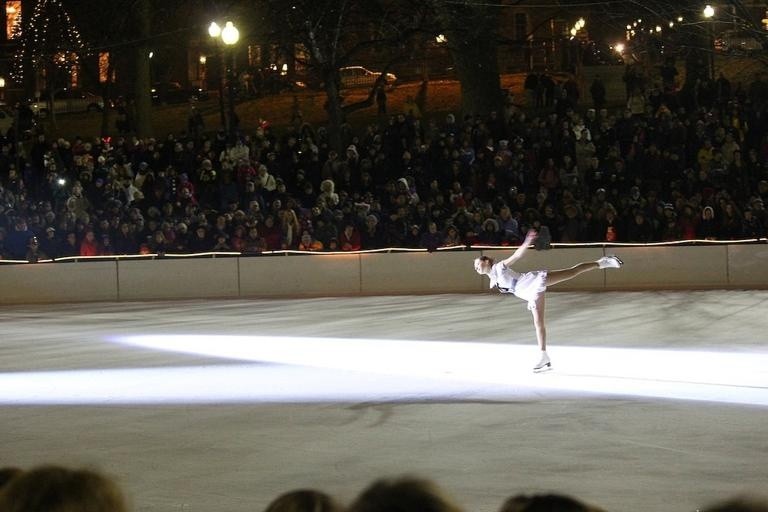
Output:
[27,88,116,121]
[113,76,205,113]
[229,68,308,99]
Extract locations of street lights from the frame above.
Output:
[703,4,717,82]
[436,34,446,79]
[208,21,240,138]
[568,14,686,101]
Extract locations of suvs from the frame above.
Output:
[714,28,766,54]
[337,65,398,90]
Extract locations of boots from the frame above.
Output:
[594,254,621,274]
[532,350,554,369]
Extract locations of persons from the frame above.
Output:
[348,473,462,511]
[263,488,347,512]
[473,230,622,371]
[499,493,605,511]
[1,464,125,511]
